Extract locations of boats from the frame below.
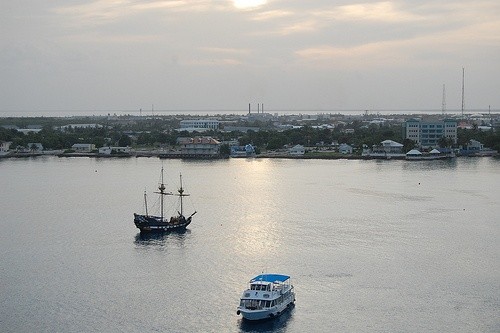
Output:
[235,267,298,321]
[134,159,198,236]
